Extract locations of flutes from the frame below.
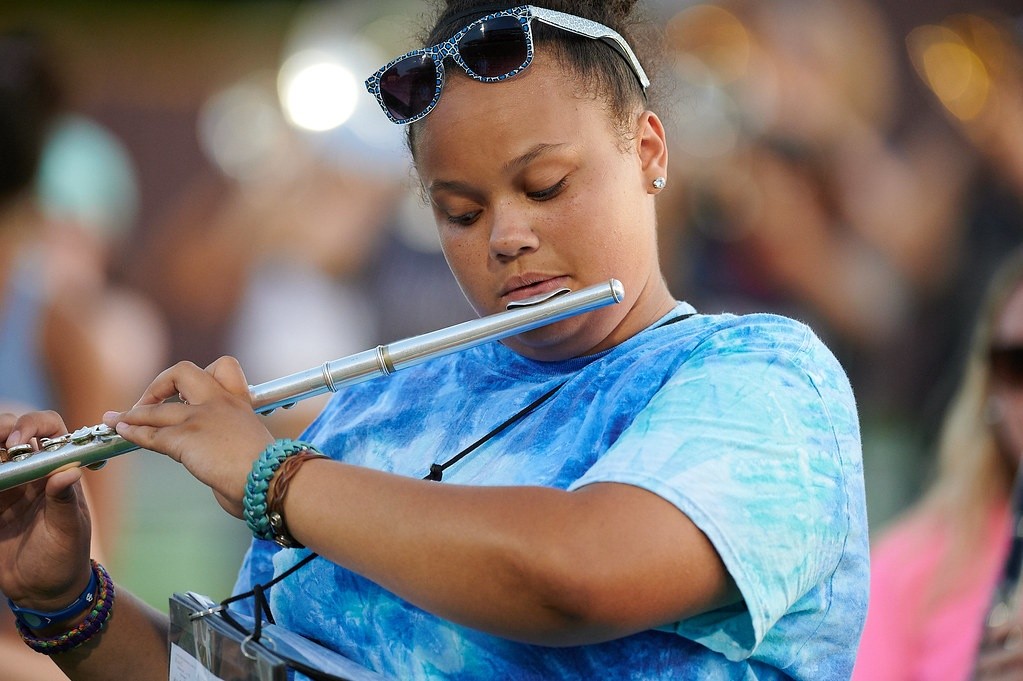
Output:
[1,280,627,489]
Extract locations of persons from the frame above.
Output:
[0,0,870,681]
[851,248,1023,681]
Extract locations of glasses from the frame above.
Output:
[986,343,1023,386]
[365,0,650,124]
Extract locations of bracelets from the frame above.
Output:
[7,559,116,655]
[242,437,331,550]
